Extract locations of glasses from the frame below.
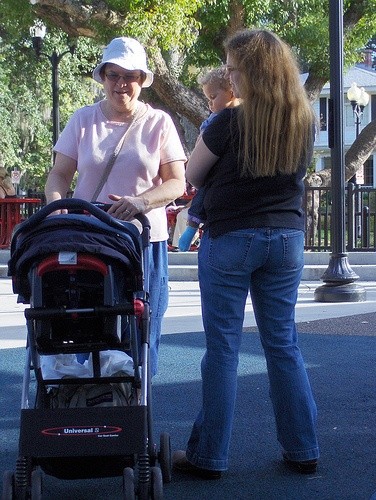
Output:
[103,69,142,82]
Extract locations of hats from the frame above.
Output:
[93,38,154,87]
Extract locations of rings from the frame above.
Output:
[125,210,130,215]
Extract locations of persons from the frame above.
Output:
[178,66,244,253]
[171,29,320,480]
[45,37,188,381]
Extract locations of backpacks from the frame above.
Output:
[47,378,140,406]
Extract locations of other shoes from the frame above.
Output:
[172,456,223,479]
[280,453,316,473]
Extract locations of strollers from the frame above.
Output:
[3,196,174,500]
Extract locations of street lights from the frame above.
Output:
[345,81,371,137]
[34,42,79,167]
